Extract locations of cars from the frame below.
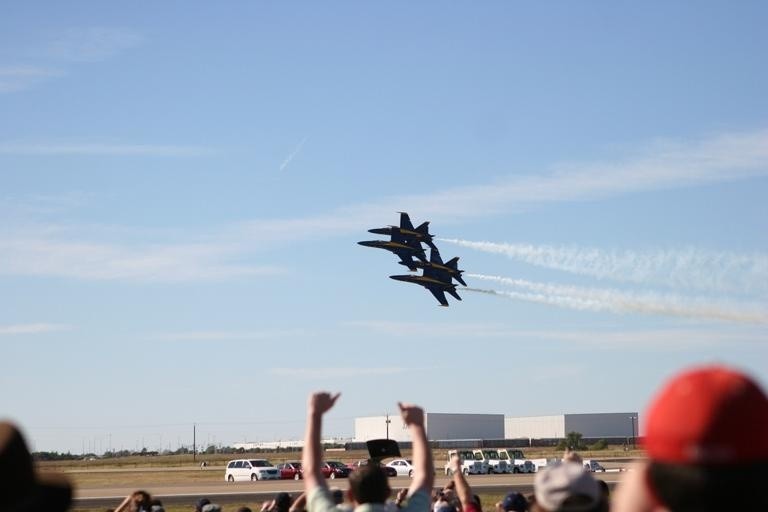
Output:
[277,463,303,480]
[385,459,412,478]
[224,458,280,481]
[444,448,535,477]
[321,461,352,479]
[346,460,397,477]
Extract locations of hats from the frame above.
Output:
[644,368,768,473]
[534,462,602,512]
[503,493,527,511]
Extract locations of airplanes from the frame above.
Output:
[356,211,467,307]
